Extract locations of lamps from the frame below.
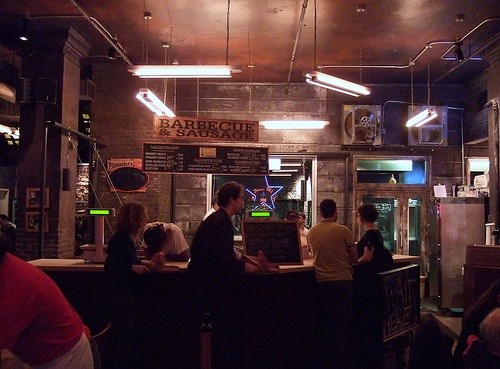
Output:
[304,69,371,98]
[136,88,175,116]
[406,109,437,129]
[127,64,232,79]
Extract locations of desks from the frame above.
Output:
[463,245,500,329]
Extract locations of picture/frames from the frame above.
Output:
[0,188,9,216]
[25,212,48,232]
[26,188,49,208]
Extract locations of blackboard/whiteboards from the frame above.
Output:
[142,142,269,176]
[241,218,303,266]
[378,264,421,342]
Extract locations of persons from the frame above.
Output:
[75,216,88,256]
[0,214,16,255]
[143,221,190,261]
[0,230,101,368]
[187,182,264,369]
[103,203,161,369]
[308,199,358,369]
[355,203,385,369]
[459,278,499,344]
[444,307,499,368]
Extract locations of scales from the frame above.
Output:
[80,208,116,263]
